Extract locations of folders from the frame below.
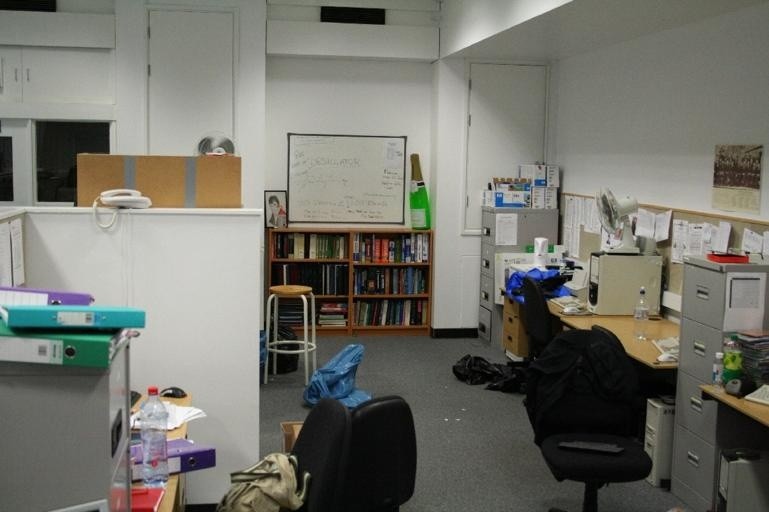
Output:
[0,305,147,328]
[130,438,216,484]
[0,287,93,306]
[1,318,132,368]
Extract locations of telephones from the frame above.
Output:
[93,189,152,213]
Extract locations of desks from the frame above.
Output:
[500,288,679,406]
[699,384,768,433]
[131,394,188,512]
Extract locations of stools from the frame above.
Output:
[263,284,317,386]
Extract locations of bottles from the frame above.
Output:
[712,350,725,392]
[723,333,746,394]
[407,154,431,229]
[633,285,650,342]
[140,386,170,488]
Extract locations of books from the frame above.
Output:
[732,328,768,406]
[267,230,431,331]
[130,487,166,512]
[112,486,130,512]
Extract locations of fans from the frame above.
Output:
[595,184,641,256]
[192,130,239,157]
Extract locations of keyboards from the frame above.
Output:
[550,296,584,309]
[131,390,141,407]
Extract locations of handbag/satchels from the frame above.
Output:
[217,451,310,512]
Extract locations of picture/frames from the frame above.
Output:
[263,189,288,228]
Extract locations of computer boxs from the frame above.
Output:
[588,253,663,317]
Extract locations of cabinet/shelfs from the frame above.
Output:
[270,227,434,337]
[0,44,114,108]
[478,205,559,345]
[668,255,768,512]
[0,334,134,512]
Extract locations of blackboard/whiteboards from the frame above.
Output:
[286,133,407,224]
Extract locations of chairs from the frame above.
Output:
[285,396,418,511]
[517,279,652,511]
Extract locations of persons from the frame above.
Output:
[266,195,288,227]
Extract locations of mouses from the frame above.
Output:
[159,387,186,398]
[563,306,579,313]
